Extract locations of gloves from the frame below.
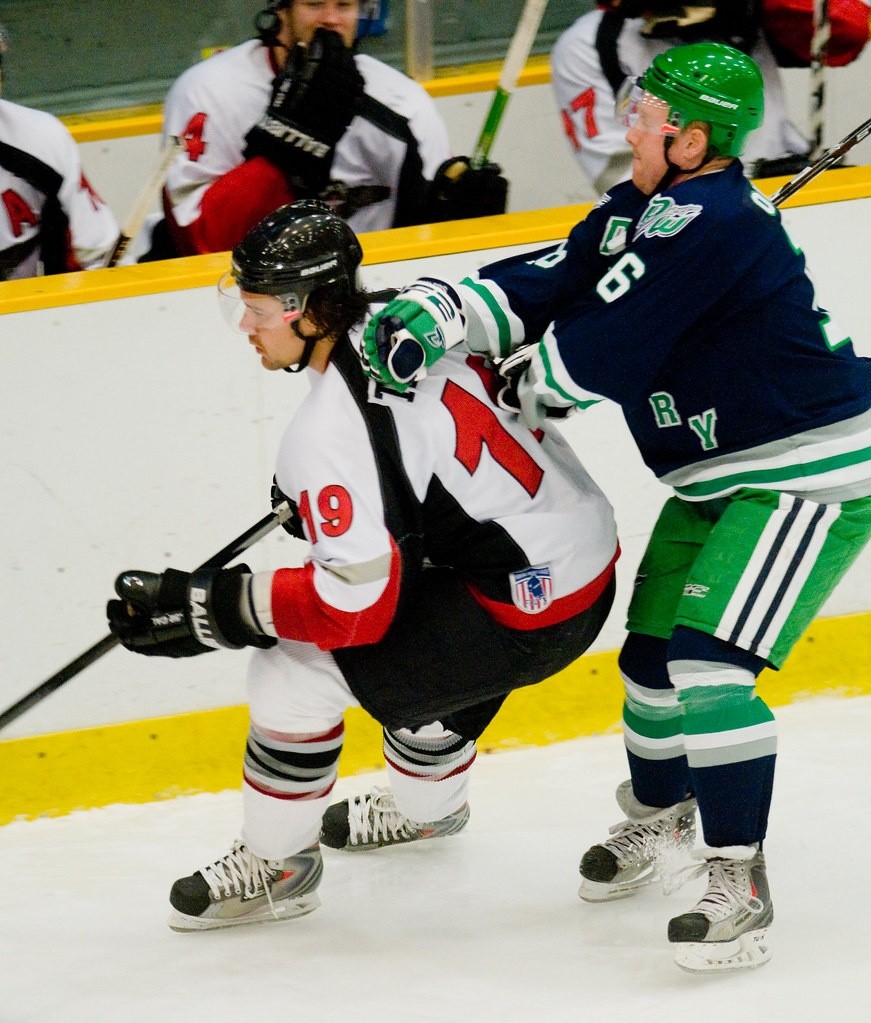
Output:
[638,0,765,55]
[496,321,605,433]
[269,474,306,542]
[428,155,510,223]
[106,562,278,659]
[241,26,365,199]
[357,269,525,394]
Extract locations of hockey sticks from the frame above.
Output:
[470,0,550,172]
[0,499,296,731]
[767,116,870,206]
[810,0,829,147]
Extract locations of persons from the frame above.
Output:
[0,99,139,283]
[550,0,871,197]
[106,201,621,930]
[122,0,508,263]
[359,40,871,973]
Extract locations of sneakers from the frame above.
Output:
[164,839,325,930]
[577,778,700,903]
[667,841,775,971]
[319,791,471,852]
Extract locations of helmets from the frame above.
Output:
[636,41,766,157]
[217,198,363,295]
